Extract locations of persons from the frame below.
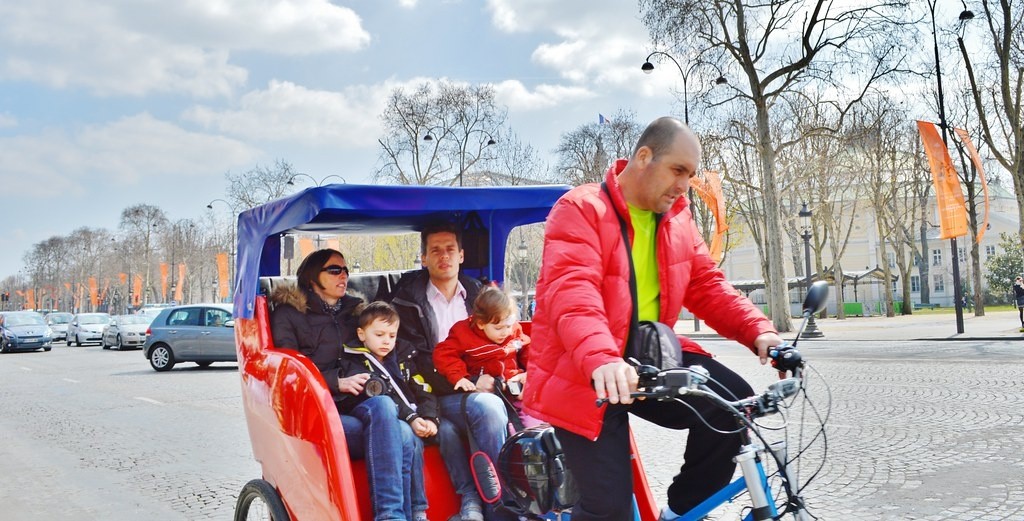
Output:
[340,301,484,521]
[387,221,529,521]
[1014,276,1024,332]
[209,315,222,327]
[270,249,414,521]
[517,295,536,321]
[961,291,968,309]
[432,288,548,435]
[521,117,785,521]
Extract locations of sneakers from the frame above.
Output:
[459,490,483,521]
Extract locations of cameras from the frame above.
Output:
[363,372,393,398]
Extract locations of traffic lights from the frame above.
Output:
[4,293,10,302]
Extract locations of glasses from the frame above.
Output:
[321,264,350,276]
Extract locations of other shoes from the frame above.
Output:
[414,512,429,521]
[658,505,715,521]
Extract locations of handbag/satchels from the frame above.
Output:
[626,320,683,371]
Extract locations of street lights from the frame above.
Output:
[152,218,195,302]
[925,0,976,333]
[641,52,727,331]
[422,125,496,187]
[798,202,824,338]
[206,199,251,302]
[287,173,347,249]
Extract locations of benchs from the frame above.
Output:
[255,270,435,461]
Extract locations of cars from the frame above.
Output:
[137,303,237,372]
[0,310,150,352]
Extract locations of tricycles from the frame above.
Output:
[234,183,830,521]
